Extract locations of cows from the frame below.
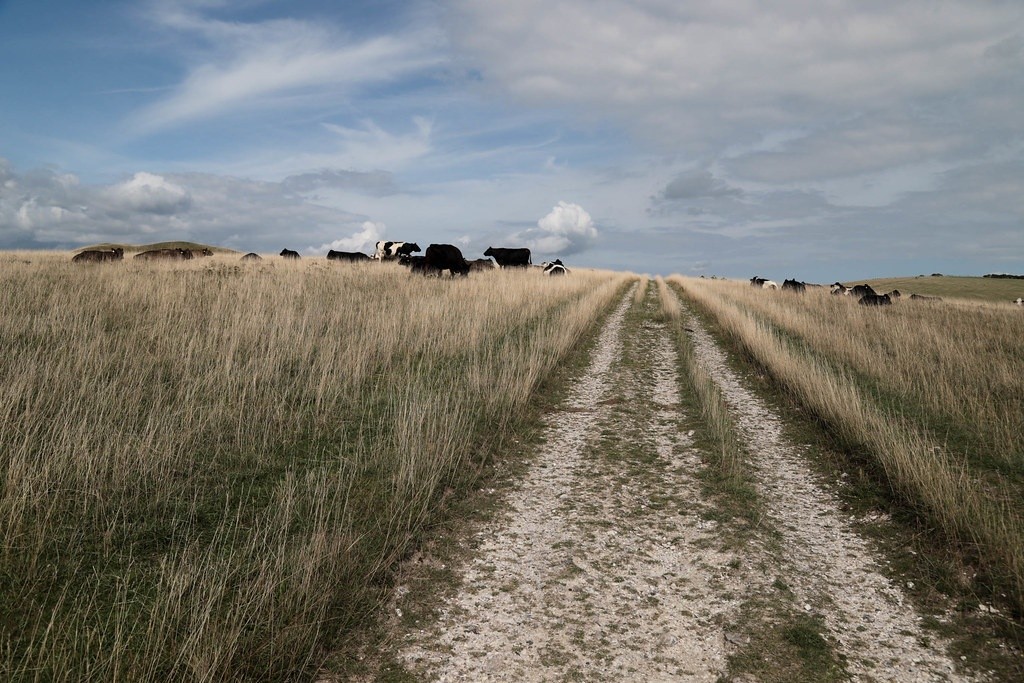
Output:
[327,241,494,279]
[544,259,571,279]
[71,247,125,263]
[483,247,534,269]
[829,281,944,306]
[132,247,263,262]
[783,278,807,294]
[1013,297,1024,307]
[280,247,302,259]
[750,275,778,291]
